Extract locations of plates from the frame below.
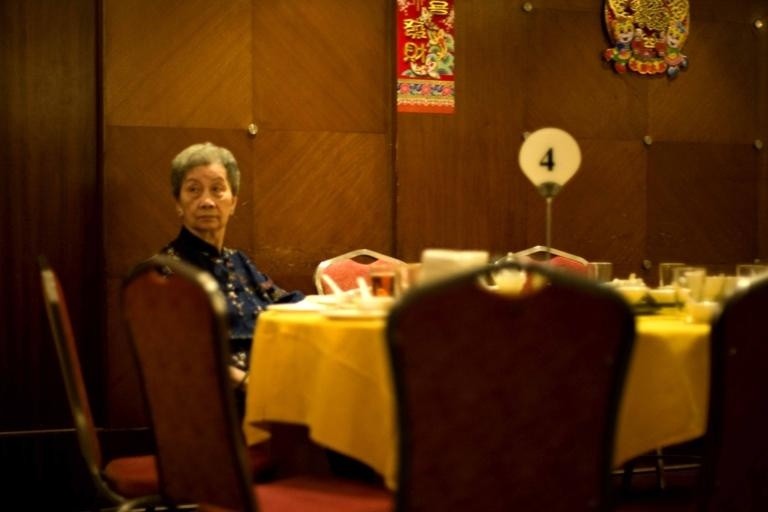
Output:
[263,291,394,320]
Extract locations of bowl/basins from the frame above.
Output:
[603,272,739,325]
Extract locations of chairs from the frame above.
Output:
[40,246,768,512]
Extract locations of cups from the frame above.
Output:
[370,261,397,299]
[585,260,613,285]
[659,263,684,290]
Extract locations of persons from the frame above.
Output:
[153,141,306,424]
